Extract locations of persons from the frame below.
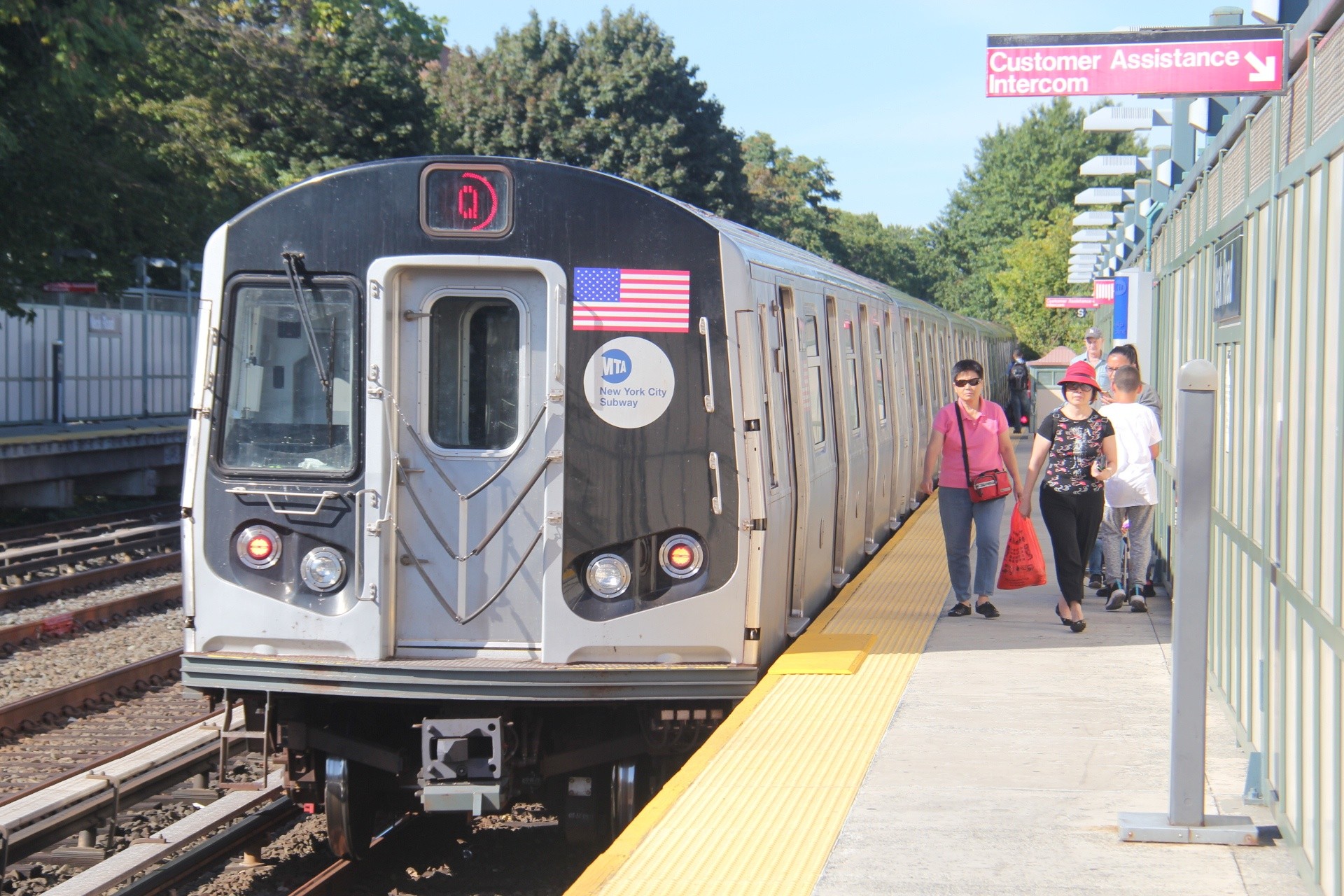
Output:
[920,360,1024,618]
[1006,349,1030,433]
[1068,328,1163,612]
[1019,361,1118,632]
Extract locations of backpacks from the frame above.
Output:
[1009,363,1026,391]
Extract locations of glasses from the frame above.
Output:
[1065,381,1092,391]
[1105,366,1119,373]
[954,376,981,386]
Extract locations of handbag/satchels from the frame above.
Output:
[969,471,1012,504]
[996,500,1047,590]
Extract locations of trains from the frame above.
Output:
[174,150,1025,869]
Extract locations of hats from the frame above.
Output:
[1058,361,1101,393]
[1085,328,1103,339]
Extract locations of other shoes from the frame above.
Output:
[1055,602,1073,625]
[1070,620,1087,632]
[1013,430,1021,434]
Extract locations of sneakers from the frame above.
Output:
[947,602,972,617]
[975,600,1001,618]
[1088,574,1156,613]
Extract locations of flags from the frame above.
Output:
[572,267,690,333]
